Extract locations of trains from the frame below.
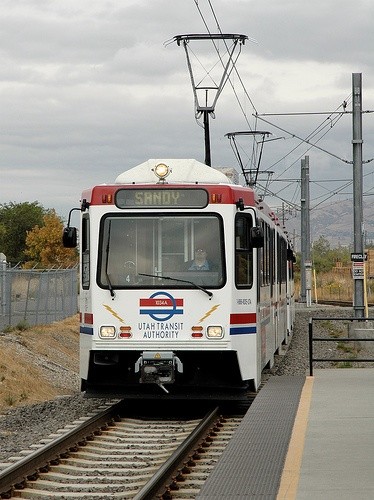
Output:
[61,156,300,404]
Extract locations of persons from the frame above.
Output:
[179,243,218,272]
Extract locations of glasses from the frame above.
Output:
[196,249,206,252]
